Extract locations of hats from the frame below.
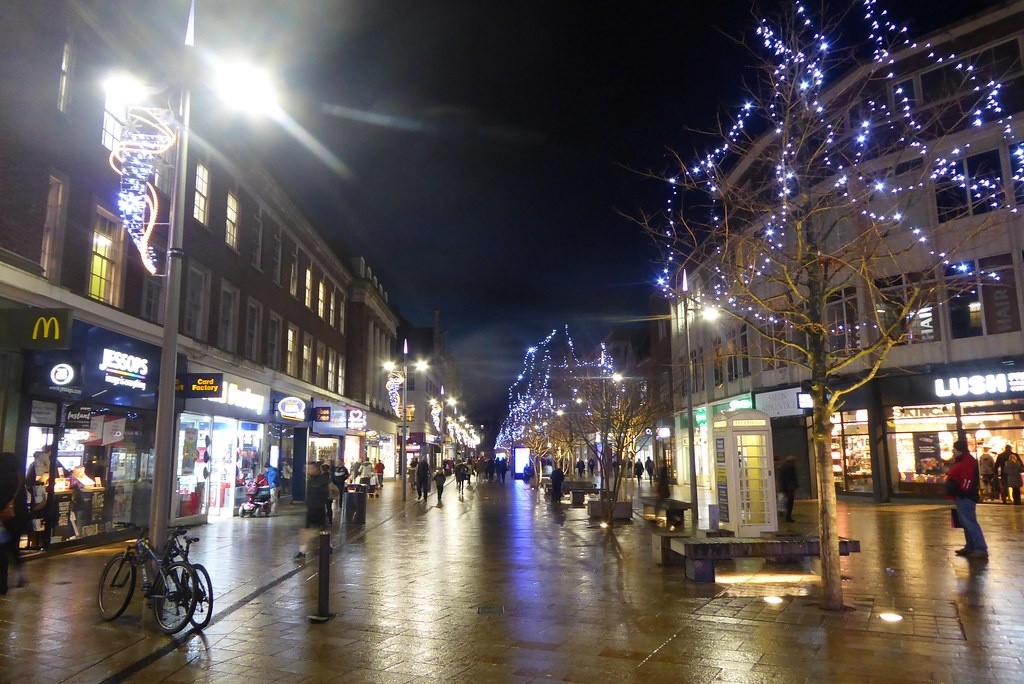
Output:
[954,440,968,452]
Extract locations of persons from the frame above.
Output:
[411,456,431,501]
[980,445,1024,505]
[656,461,669,520]
[524,463,534,480]
[577,458,585,478]
[292,458,348,561]
[550,467,564,503]
[465,456,508,485]
[443,458,469,496]
[27,445,67,503]
[947,441,988,558]
[434,468,446,501]
[777,452,800,523]
[264,463,279,516]
[0,452,34,594]
[635,459,644,485]
[588,458,595,475]
[350,458,385,487]
[645,457,656,482]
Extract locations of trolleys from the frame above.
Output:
[238,478,274,518]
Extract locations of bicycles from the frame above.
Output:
[167,523,215,630]
[97,519,199,635]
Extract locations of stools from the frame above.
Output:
[696,529,735,537]
[569,490,585,508]
[598,490,617,502]
[652,532,689,564]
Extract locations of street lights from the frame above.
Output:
[446,406,467,466]
[429,384,457,474]
[384,338,428,502]
[669,269,721,525]
[596,352,625,521]
[94,0,275,622]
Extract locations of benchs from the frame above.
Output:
[639,496,691,530]
[669,534,860,582]
[545,481,633,519]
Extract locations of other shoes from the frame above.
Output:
[786,519,795,522]
[968,550,988,559]
[955,546,973,554]
[328,524,332,526]
[418,494,422,500]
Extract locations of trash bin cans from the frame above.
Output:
[420,474,431,492]
[341,484,368,522]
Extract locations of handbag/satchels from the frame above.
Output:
[950,509,962,528]
[0,500,16,524]
[328,475,340,500]
